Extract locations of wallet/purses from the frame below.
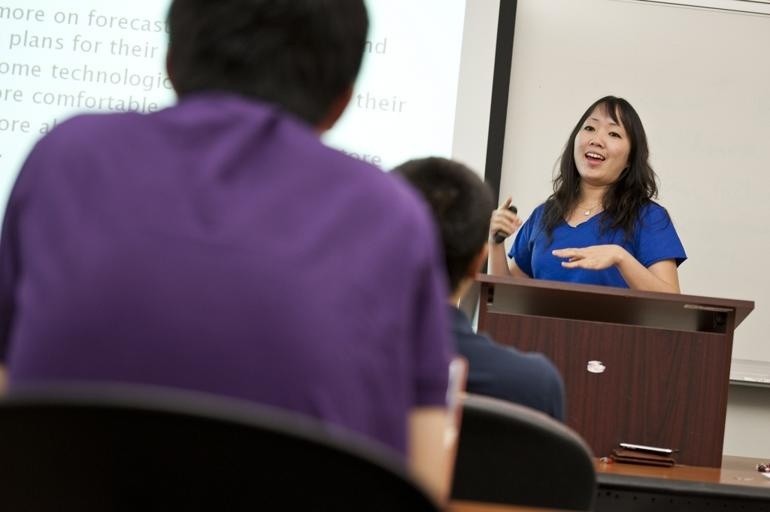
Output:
[611,445,675,467]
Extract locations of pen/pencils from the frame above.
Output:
[759,464,770,472]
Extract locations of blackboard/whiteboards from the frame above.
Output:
[479,0,770,387]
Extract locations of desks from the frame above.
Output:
[588,453,770,511]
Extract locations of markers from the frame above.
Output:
[495,206,517,243]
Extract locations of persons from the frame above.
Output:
[485,92,689,295]
[384,152,570,429]
[1,1,455,512]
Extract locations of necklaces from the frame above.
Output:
[576,196,607,218]
